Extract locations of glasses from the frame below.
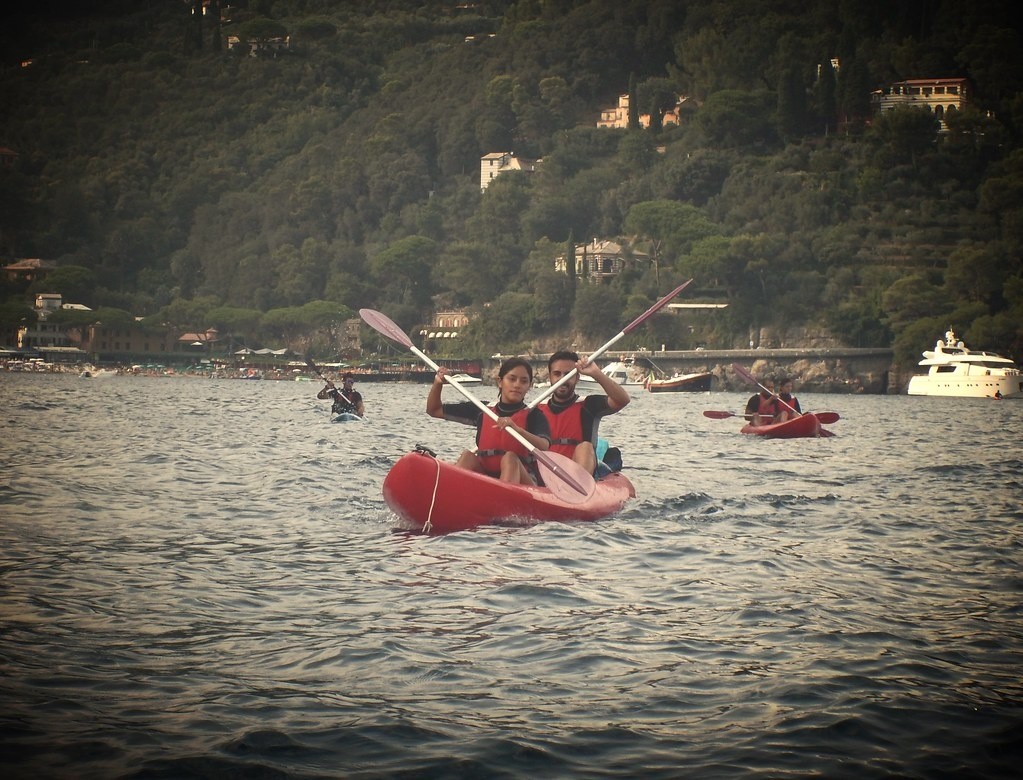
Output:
[344,380,354,383]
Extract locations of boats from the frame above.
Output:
[579,361,629,384]
[740,413,821,438]
[450,375,483,387]
[329,413,366,422]
[381,443,636,535]
[643,371,716,392]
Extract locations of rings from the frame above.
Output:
[504,419,507,422]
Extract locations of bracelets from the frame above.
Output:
[325,385,329,390]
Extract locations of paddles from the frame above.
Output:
[309,361,362,416]
[360,308,595,501]
[703,410,841,424]
[529,274,694,413]
[733,364,802,417]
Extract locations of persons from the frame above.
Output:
[316,371,364,420]
[530,350,630,479]
[761,378,802,426]
[744,377,780,427]
[425,355,550,487]
